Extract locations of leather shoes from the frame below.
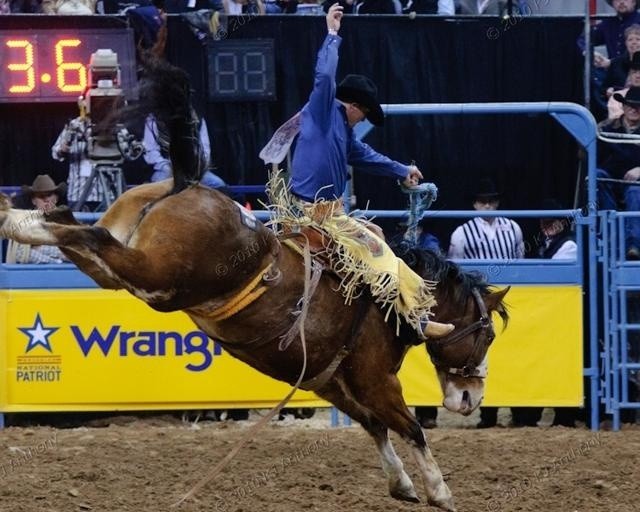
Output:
[417,418,436,428]
[414,319,456,346]
[477,418,495,427]
[628,249,640,261]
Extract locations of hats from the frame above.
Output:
[538,195,566,209]
[336,74,385,126]
[621,52,640,71]
[469,171,500,201]
[613,86,640,108]
[23,175,68,202]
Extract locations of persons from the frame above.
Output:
[605,52,639,122]
[0,1,503,17]
[395,212,443,430]
[597,86,640,261]
[140,107,225,188]
[1,172,72,265]
[445,180,528,430]
[599,20,639,99]
[525,201,576,430]
[277,3,458,345]
[576,0,638,68]
[54,88,143,210]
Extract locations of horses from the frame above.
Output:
[0,57,512,511]
